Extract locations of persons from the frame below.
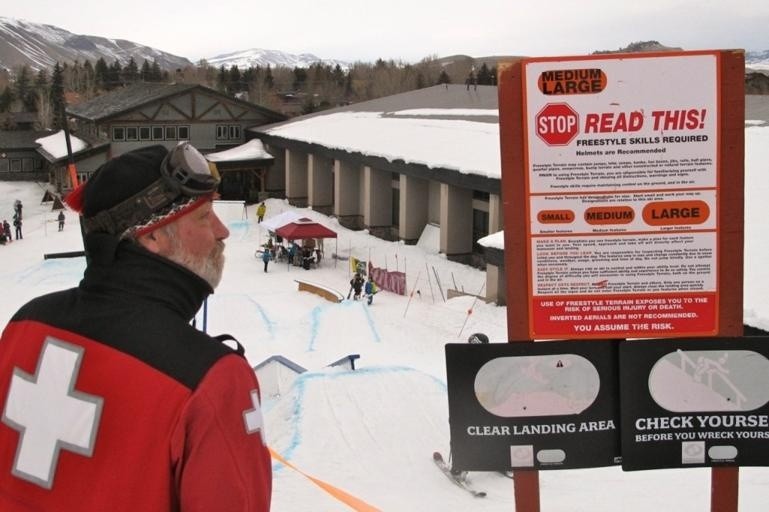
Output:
[57,211,65,232]
[267,238,322,270]
[255,202,266,224]
[262,248,272,272]
[363,276,378,305]
[0,199,24,245]
[0,143,278,511]
[351,273,365,300]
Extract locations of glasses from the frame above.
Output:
[163,140,223,194]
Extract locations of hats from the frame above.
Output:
[79,147,220,242]
